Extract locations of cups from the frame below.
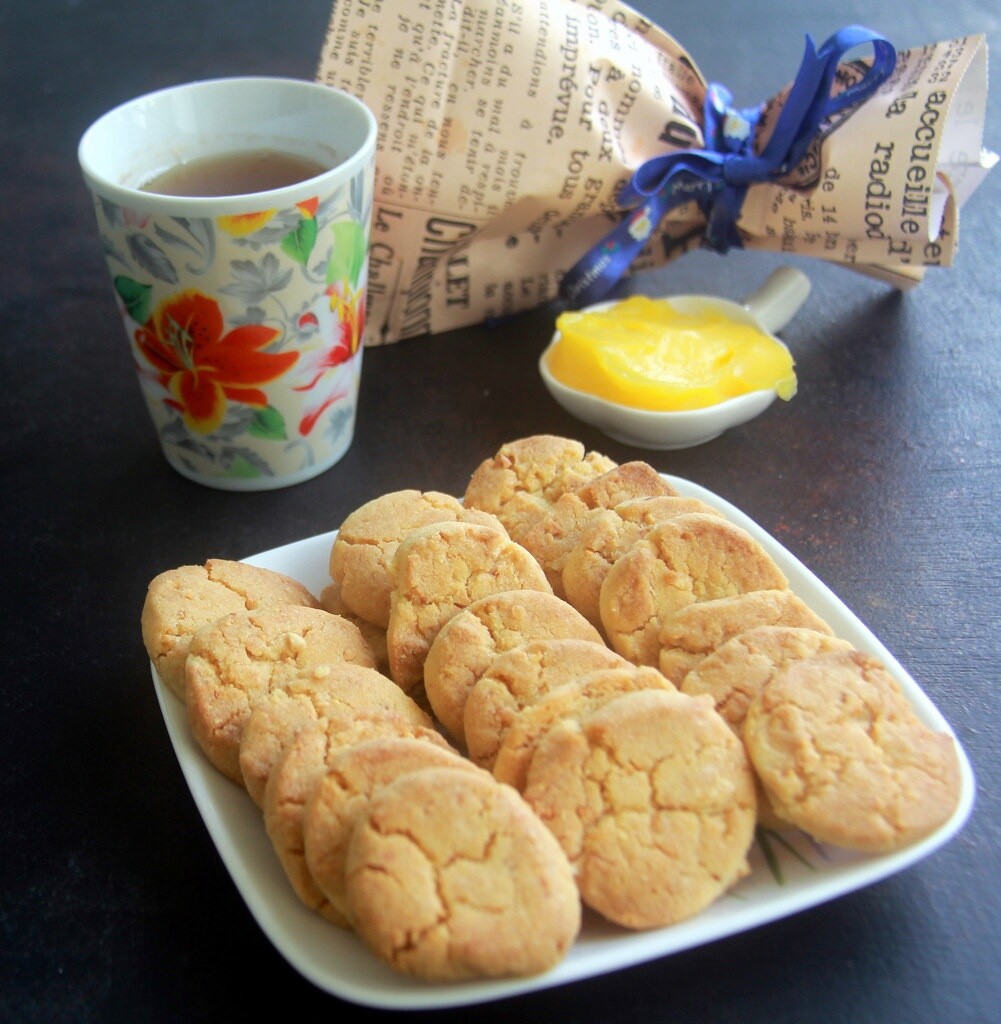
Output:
[75,74,378,491]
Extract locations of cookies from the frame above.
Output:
[141,435,962,982]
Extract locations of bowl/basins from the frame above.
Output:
[536,262,810,454]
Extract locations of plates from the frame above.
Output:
[148,473,976,1009]
